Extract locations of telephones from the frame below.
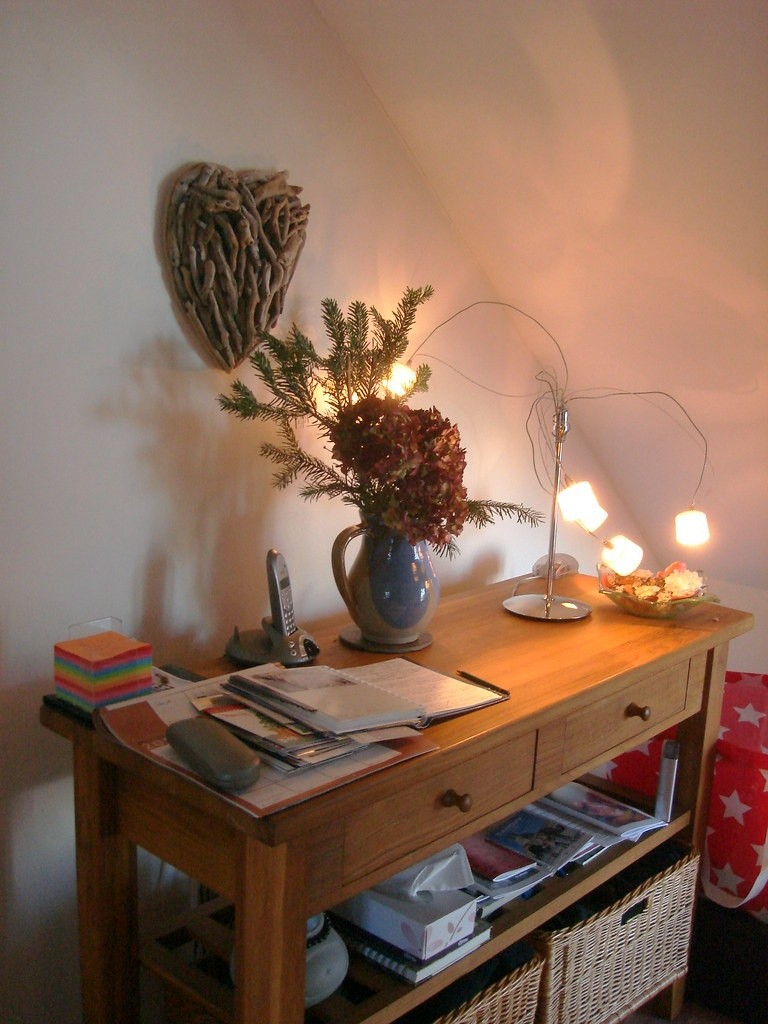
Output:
[224,545,321,667]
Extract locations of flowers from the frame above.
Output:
[218,284,546,561]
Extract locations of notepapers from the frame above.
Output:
[54,630,154,715]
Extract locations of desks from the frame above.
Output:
[38,572,754,1024]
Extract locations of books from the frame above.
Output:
[326,782,671,986]
[217,654,510,740]
[188,662,368,773]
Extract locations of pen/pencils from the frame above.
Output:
[455,669,511,699]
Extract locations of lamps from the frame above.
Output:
[384,300,711,622]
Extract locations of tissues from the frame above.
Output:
[324,842,480,962]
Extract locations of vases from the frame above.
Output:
[331,507,440,653]
